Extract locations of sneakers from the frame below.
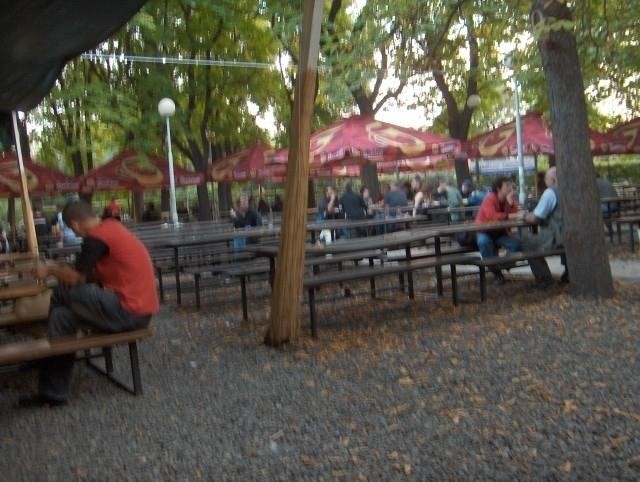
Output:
[19,395,69,408]
[495,275,570,288]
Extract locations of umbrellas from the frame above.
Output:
[204,137,290,201]
[79,145,208,222]
[462,108,629,199]
[266,113,460,189]
[0,147,82,244]
[606,116,640,155]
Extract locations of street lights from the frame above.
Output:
[504,54,527,209]
[466,93,484,186]
[153,95,183,228]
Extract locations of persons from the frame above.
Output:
[473,174,520,287]
[1,198,83,254]
[104,197,120,219]
[229,195,282,249]
[595,171,621,213]
[17,197,160,408]
[522,166,566,288]
[143,201,159,221]
[314,175,487,240]
